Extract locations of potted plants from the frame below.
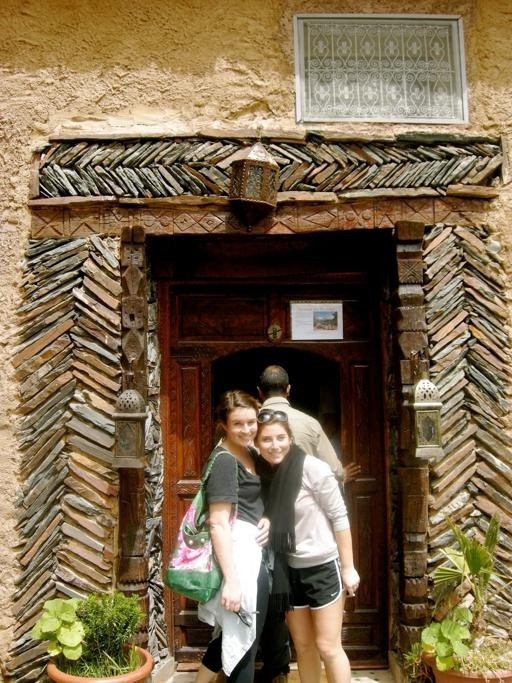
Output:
[402,511,511,683]
[31,587,155,683]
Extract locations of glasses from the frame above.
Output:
[235,606,252,628]
[257,412,288,425]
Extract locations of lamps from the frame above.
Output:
[227,123,281,226]
[111,358,148,469]
[406,349,445,458]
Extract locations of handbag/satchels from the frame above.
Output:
[167,452,240,602]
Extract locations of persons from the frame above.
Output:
[258,362,362,683]
[255,408,362,682]
[186,390,277,683]
